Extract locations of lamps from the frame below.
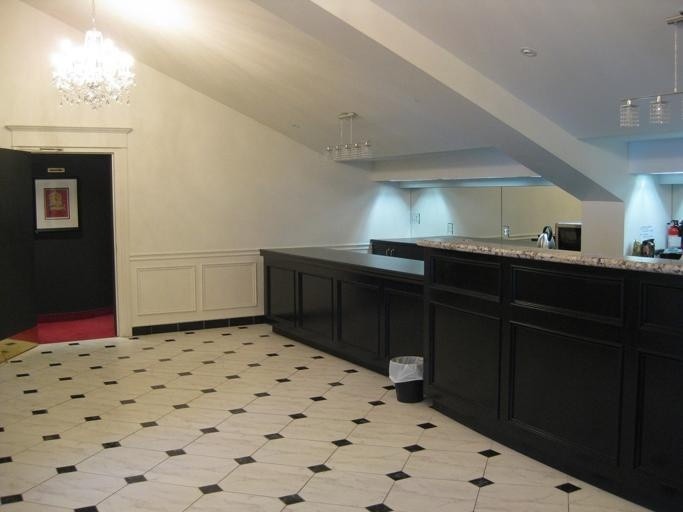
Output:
[611,8,681,129]
[49,3,136,119]
[321,111,375,164]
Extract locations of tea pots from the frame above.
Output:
[536,225,555,250]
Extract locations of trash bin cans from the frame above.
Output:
[389,355,424,403]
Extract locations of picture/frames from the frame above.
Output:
[30,175,81,233]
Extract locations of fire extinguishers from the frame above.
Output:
[667,220,680,248]
